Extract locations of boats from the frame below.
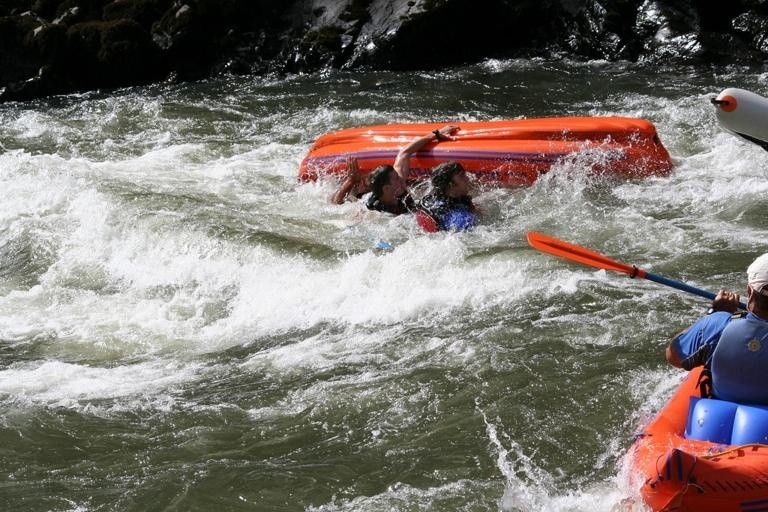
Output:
[617,365,768,510]
[296,116,672,196]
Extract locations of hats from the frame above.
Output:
[746,252,768,296]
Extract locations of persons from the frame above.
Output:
[665,253,768,408]
[368,125,461,215]
[418,162,476,231]
[332,155,366,204]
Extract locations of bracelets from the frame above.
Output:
[432,129,444,141]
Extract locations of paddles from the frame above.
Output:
[526,229,747,310]
